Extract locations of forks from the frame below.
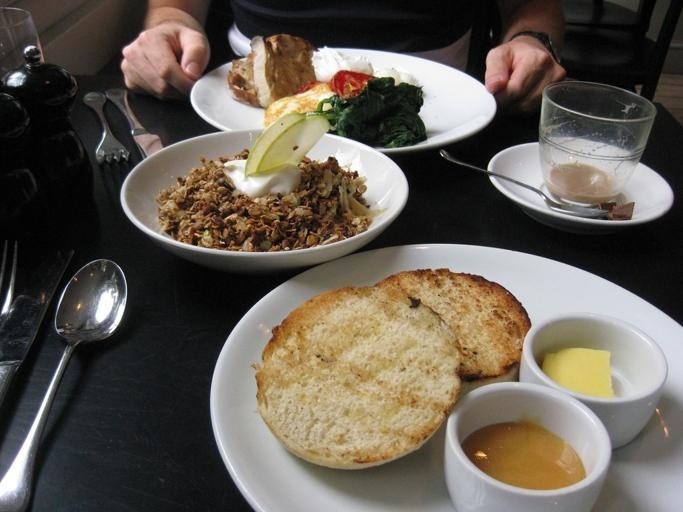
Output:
[0,236,19,326]
[82,91,131,167]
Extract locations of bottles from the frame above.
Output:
[0,45,95,205]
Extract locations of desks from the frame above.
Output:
[0,69,683,511]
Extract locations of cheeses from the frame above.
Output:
[543,347,615,399]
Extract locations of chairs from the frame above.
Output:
[498,0,683,103]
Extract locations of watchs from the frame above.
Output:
[509,31,564,64]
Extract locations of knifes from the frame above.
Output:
[0,247,74,408]
[106,86,162,162]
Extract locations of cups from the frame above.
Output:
[538,82,656,210]
[442,379,611,511]
[0,5,43,81]
[519,313,670,449]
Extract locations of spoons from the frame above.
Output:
[0,258,130,511]
[436,148,609,219]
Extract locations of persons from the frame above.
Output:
[119,0,567,121]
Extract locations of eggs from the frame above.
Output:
[264,83,331,127]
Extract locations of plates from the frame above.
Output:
[118,126,410,268]
[205,242,682,512]
[486,137,675,237]
[189,46,498,152]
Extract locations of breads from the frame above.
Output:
[255,284,462,472]
[375,269,531,379]
